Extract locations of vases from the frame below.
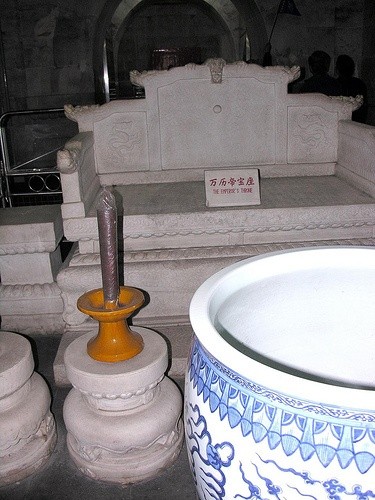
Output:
[183,245,375,500]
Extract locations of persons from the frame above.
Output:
[299,51,342,96]
[335,54,369,123]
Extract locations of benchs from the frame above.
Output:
[57,59,375,327]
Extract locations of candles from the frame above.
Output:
[97,197,118,301]
